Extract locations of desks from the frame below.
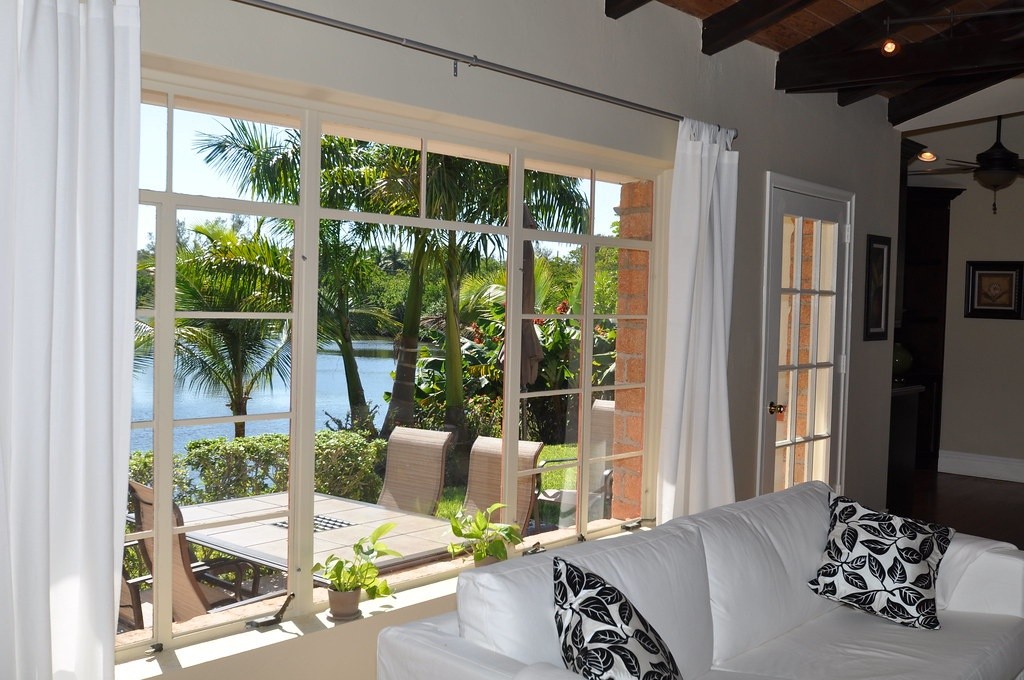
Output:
[126,490,512,587]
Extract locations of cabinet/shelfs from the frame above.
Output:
[892,384,927,472]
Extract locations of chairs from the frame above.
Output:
[534,399,615,534]
[129,481,289,630]
[377,427,453,516]
[455,435,544,536]
[119,540,243,630]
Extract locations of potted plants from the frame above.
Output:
[447,503,525,567]
[310,521,403,619]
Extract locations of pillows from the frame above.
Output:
[807,492,956,630]
[553,556,683,680]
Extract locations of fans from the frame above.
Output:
[907,115,1024,176]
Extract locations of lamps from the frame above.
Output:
[881,16,900,57]
[973,171,1018,216]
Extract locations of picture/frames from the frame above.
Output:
[964,260,1024,320]
[863,234,892,341]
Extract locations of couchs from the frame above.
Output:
[377,481,1024,680]
